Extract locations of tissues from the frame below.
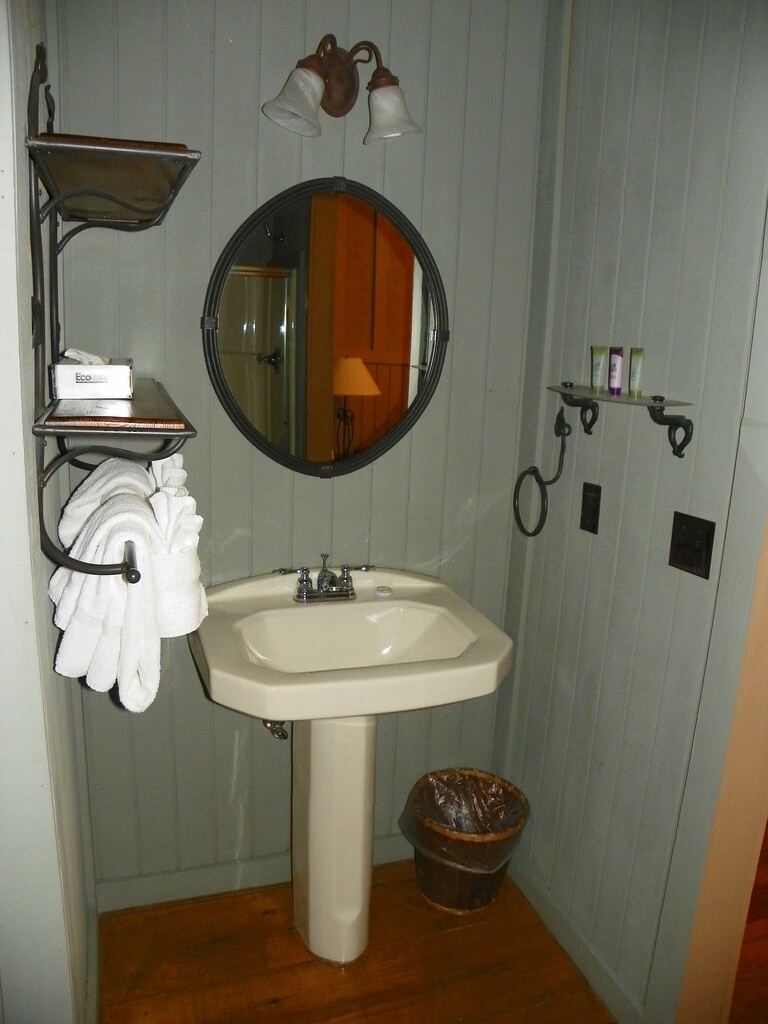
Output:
[51,348,134,401]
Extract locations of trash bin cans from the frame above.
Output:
[409,769,531,916]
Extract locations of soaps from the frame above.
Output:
[375,585,393,597]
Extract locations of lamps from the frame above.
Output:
[261,33,425,147]
[333,355,381,461]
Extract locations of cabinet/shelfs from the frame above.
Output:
[307,192,414,462]
[24,43,202,584]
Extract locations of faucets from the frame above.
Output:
[317,569,341,602]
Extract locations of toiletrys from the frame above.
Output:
[606,346,624,395]
[629,347,646,398]
[589,346,608,395]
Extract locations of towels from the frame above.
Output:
[51,454,207,715]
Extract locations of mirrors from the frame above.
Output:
[199,175,450,478]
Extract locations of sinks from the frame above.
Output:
[191,565,511,721]
